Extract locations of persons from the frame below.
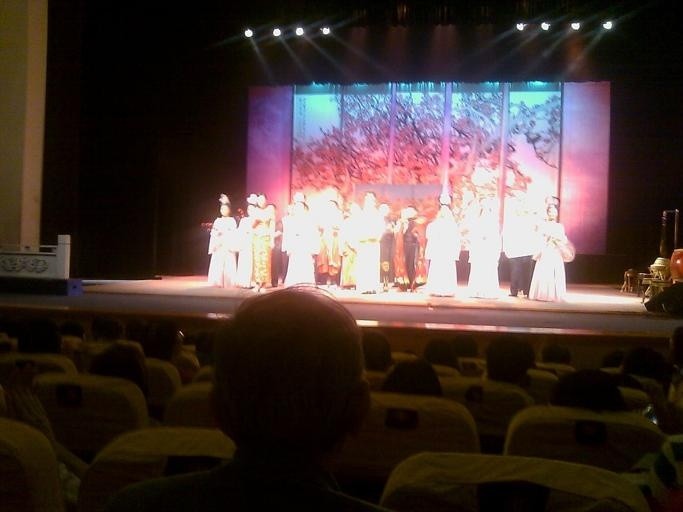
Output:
[198,184,579,303]
[0,276,683,512]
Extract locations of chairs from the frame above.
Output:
[1,335,681,509]
